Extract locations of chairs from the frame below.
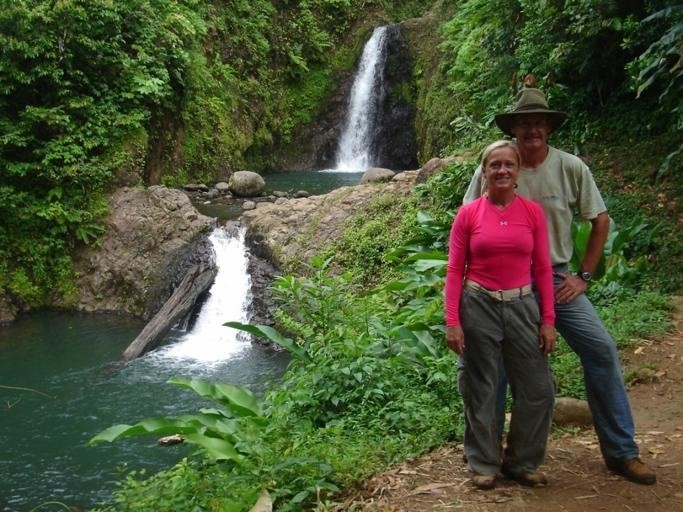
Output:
[605,456,656,486]
[463,446,548,489]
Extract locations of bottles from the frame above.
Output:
[494,90,566,136]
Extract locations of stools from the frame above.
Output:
[463,277,532,301]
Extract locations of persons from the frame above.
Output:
[461,87,660,485]
[445,139,558,488]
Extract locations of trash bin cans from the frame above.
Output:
[577,270,594,283]
